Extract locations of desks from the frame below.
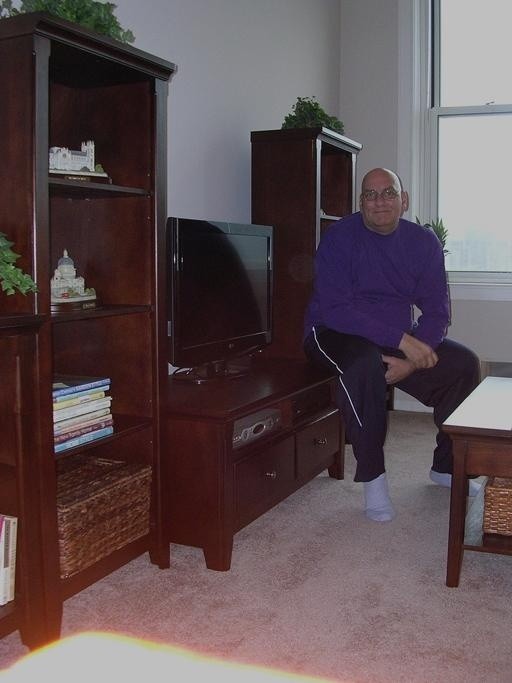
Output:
[442,370,510,590]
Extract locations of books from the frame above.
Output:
[0,514,18,607]
[51,371,115,455]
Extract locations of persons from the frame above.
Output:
[302,166,482,523]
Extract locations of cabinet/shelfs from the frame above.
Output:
[169,358,347,572]
[0,8,180,650]
[247,123,364,356]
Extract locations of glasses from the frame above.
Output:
[362,187,403,201]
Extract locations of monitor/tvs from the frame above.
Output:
[166,216,275,385]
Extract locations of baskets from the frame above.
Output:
[55,453,151,580]
[482,477,512,537]
